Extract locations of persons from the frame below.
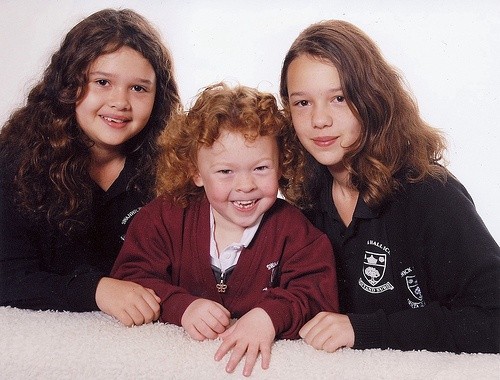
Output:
[110,82,340,378]
[0,9,187,327]
[276,19,499,354]
